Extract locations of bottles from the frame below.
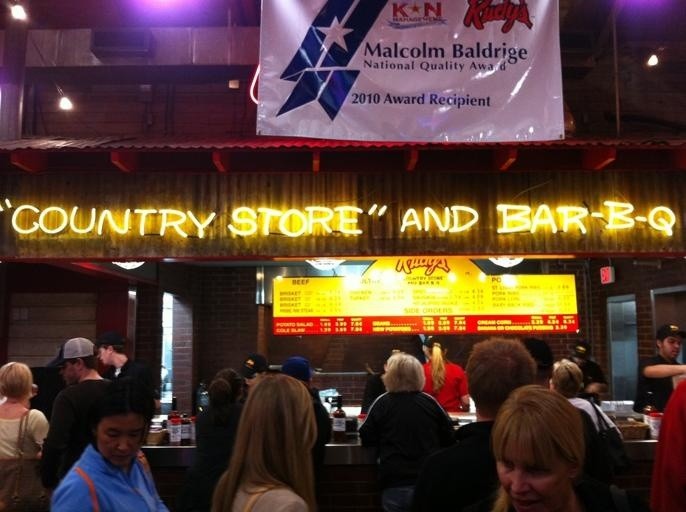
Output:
[168,395,197,447]
[332,395,348,444]
[643,405,664,438]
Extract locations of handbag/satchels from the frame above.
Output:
[598,427,631,475]
[0,457,52,507]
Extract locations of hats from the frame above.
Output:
[241,354,265,378]
[424,335,448,349]
[657,324,685,341]
[282,357,315,378]
[575,342,590,357]
[94,334,118,345]
[48,337,96,367]
